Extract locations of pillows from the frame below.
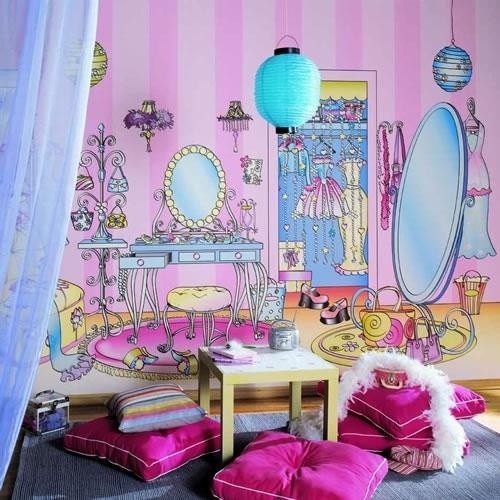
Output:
[62,416,222,483]
[211,430,389,500]
[341,371,487,442]
[105,382,207,433]
[339,410,471,459]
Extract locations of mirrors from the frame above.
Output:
[163,143,226,230]
[390,100,469,304]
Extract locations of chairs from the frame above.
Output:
[0,202,86,366]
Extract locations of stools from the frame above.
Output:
[197,344,339,464]
[156,286,234,351]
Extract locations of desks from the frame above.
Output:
[118,239,269,341]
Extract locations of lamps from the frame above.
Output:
[122,99,175,153]
[217,100,252,153]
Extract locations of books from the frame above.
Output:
[206,346,258,365]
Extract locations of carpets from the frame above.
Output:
[75,315,273,381]
[13,410,500,499]
[311,318,478,370]
